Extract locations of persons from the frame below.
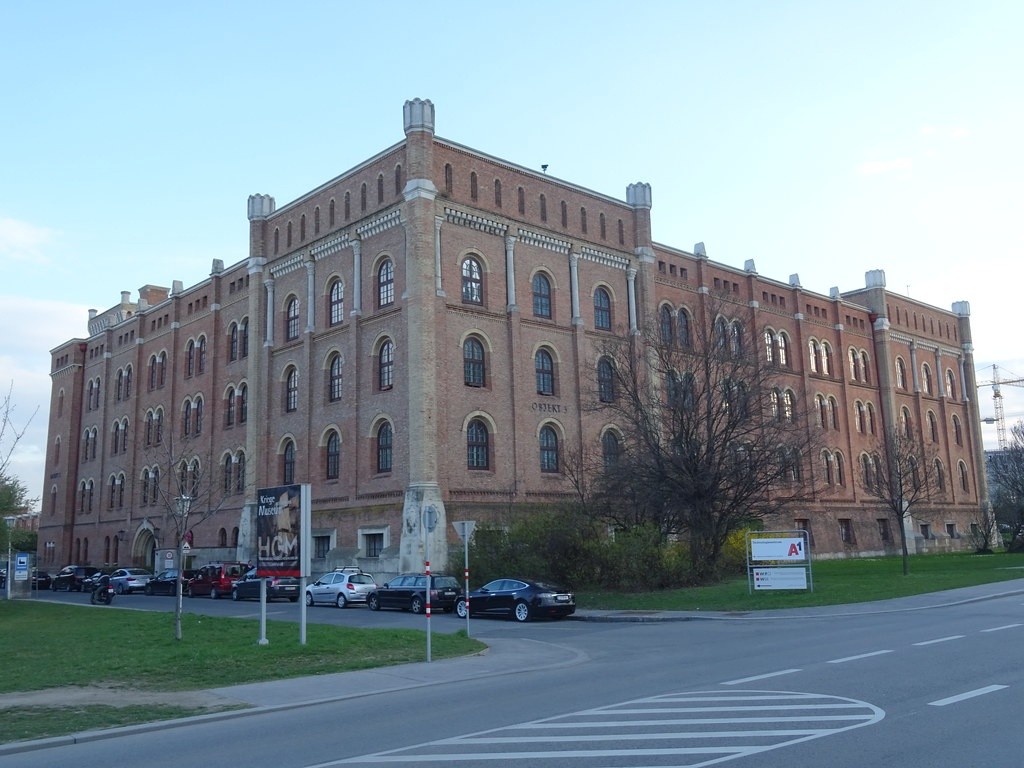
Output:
[92,570,109,600]
[155,571,157,579]
[244,560,255,574]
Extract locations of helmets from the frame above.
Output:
[100,568,107,573]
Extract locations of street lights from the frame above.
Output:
[3,516,17,600]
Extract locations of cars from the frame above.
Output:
[453,575,576,623]
[32,570,52,590]
[110,567,156,596]
[50,564,97,593]
[231,567,301,603]
[0,568,6,588]
[144,568,198,597]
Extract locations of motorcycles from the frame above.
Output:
[85,570,116,606]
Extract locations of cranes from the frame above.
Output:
[976,364,1023,453]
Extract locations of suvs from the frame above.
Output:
[187,562,255,599]
[365,572,463,614]
[306,565,380,609]
